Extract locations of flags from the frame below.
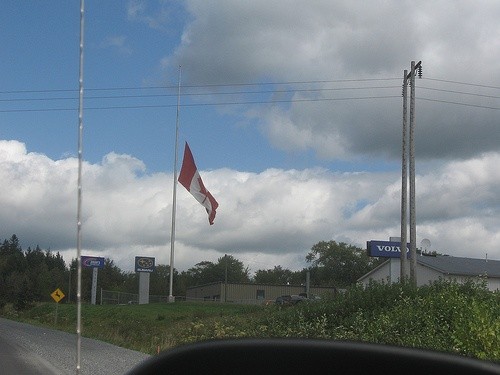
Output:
[177,137,219,226]
